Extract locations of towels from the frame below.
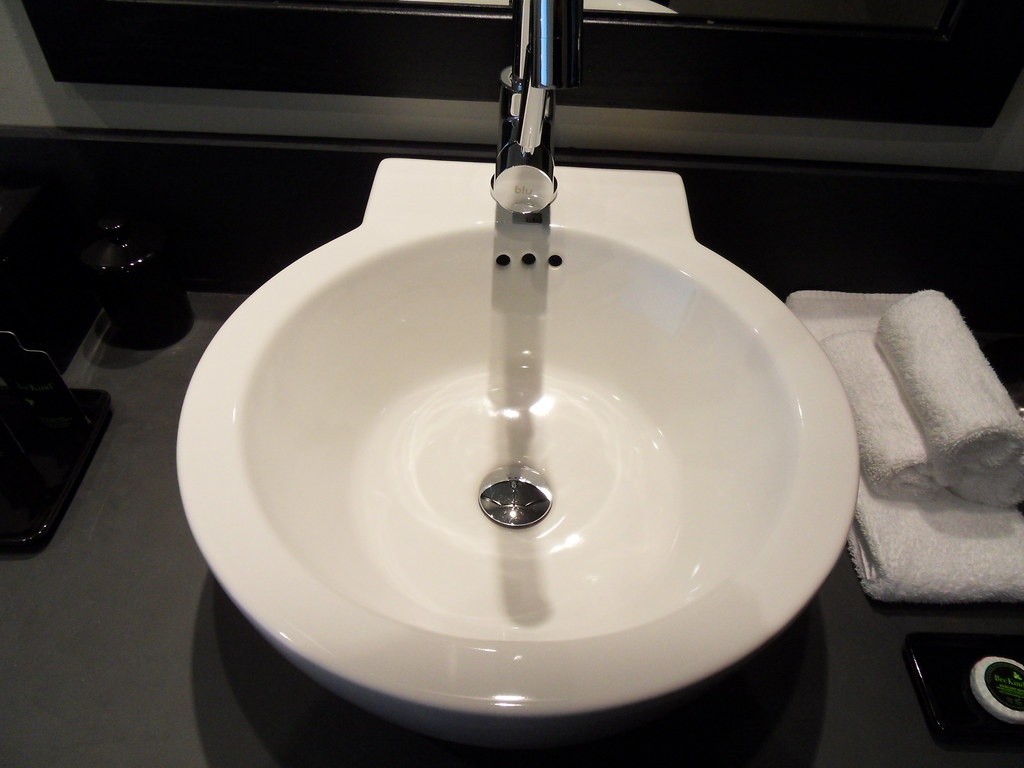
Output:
[784,285,1023,605]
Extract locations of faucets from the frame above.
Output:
[489,0,584,225]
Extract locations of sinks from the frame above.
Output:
[172,153,865,749]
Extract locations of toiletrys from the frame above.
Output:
[96,213,195,350]
